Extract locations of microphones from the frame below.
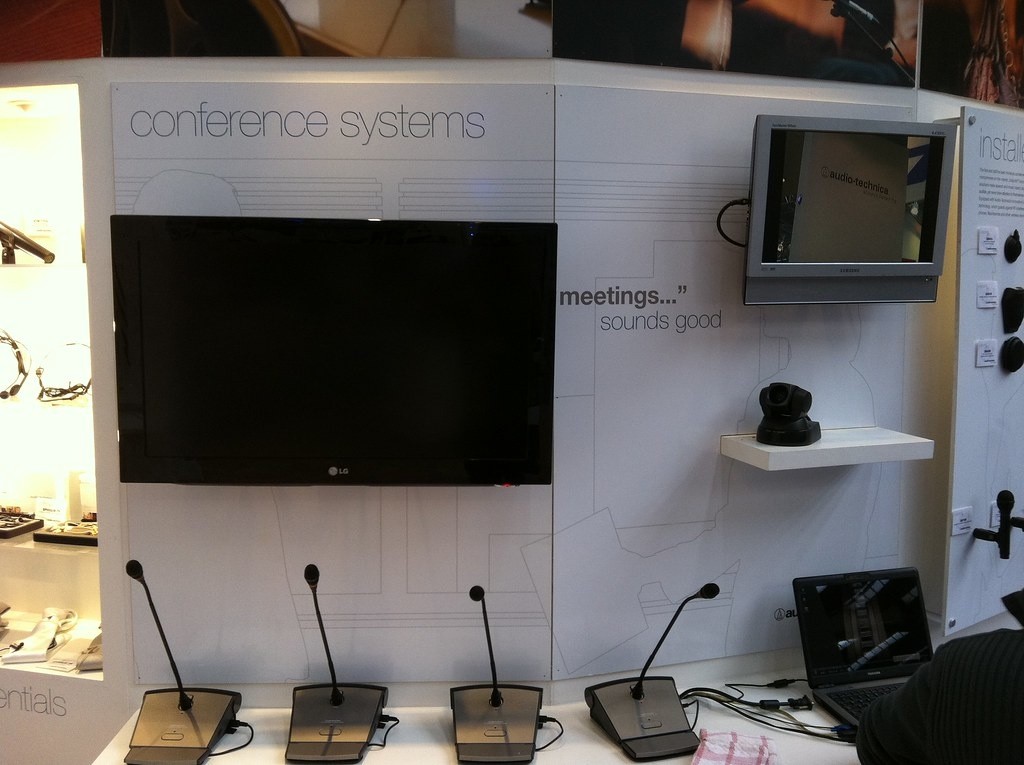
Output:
[449,584,544,765]
[285,564,390,765]
[122,560,241,765]
[584,583,720,763]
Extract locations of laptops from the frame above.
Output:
[791,567,935,727]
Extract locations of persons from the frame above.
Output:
[853,627,1024,765]
[552,0,915,87]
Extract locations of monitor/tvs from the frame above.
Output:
[111,215,558,488]
[744,115,957,307]
